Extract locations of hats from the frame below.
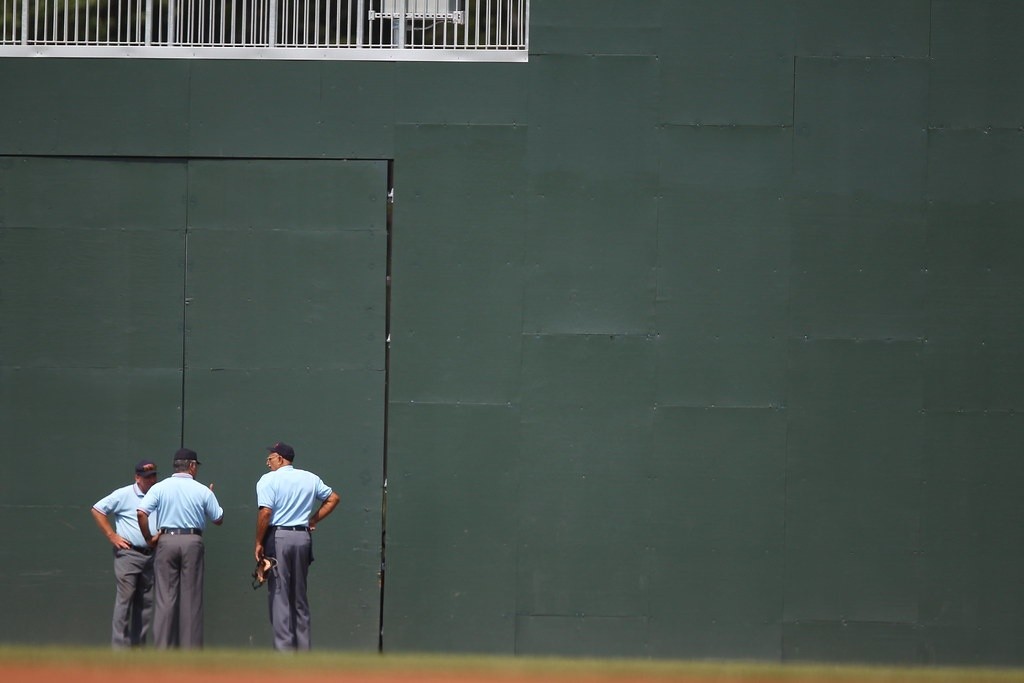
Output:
[135,460,159,477]
[175,448,202,465]
[267,442,294,462]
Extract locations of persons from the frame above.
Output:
[137,448,224,651]
[256,442,339,650]
[91,461,160,648]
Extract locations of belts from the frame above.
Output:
[158,528,202,536]
[267,526,309,532]
[125,543,154,555]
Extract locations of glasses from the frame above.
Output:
[268,454,281,461]
